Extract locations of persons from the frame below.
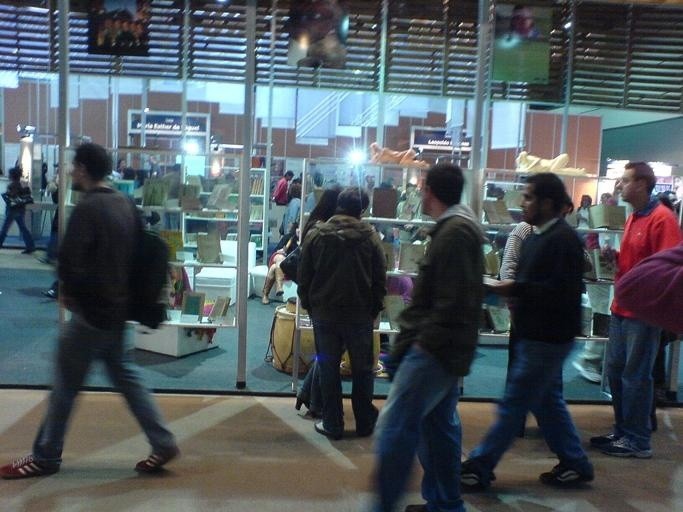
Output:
[506,5,550,43]
[279,186,378,436]
[272,143,681,281]
[591,163,682,458]
[98,17,145,48]
[1,141,181,474]
[364,164,484,511]
[2,143,182,297]
[289,0,347,67]
[461,173,594,482]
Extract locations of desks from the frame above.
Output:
[183,215,263,260]
[125,315,237,357]
[22,203,61,245]
[271,305,378,380]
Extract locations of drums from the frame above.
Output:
[270,304,331,375]
[285,298,308,314]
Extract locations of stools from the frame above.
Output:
[251,262,295,304]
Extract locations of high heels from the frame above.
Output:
[295,397,311,412]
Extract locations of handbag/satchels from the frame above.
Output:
[279,245,300,287]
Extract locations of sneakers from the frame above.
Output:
[539,462,594,486]
[0,453,58,480]
[459,465,497,492]
[315,419,344,438]
[405,504,427,511]
[356,408,378,437]
[136,441,181,474]
[591,432,653,458]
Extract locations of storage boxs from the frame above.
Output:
[589,204,625,230]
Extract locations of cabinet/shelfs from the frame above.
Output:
[296,156,625,339]
[63,145,182,258]
[197,166,264,224]
[482,180,525,226]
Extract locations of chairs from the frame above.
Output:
[194,240,256,306]
[398,243,425,273]
[376,271,414,343]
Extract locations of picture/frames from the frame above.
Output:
[207,295,232,319]
[181,290,206,316]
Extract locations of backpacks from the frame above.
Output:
[131,227,173,330]
